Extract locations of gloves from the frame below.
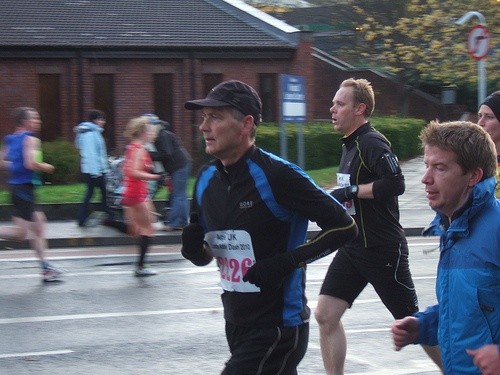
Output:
[243,253,293,293]
[182,213,206,254]
[327,185,358,203]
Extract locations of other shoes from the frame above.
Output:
[135,268,157,276]
[42,265,66,280]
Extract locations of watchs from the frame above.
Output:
[351,185,359,199]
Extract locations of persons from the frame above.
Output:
[180,80,359,375]
[311,79,443,375]
[0,105,194,283]
[390,120,500,374]
[477,90,500,202]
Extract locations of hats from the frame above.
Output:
[185,80,261,127]
